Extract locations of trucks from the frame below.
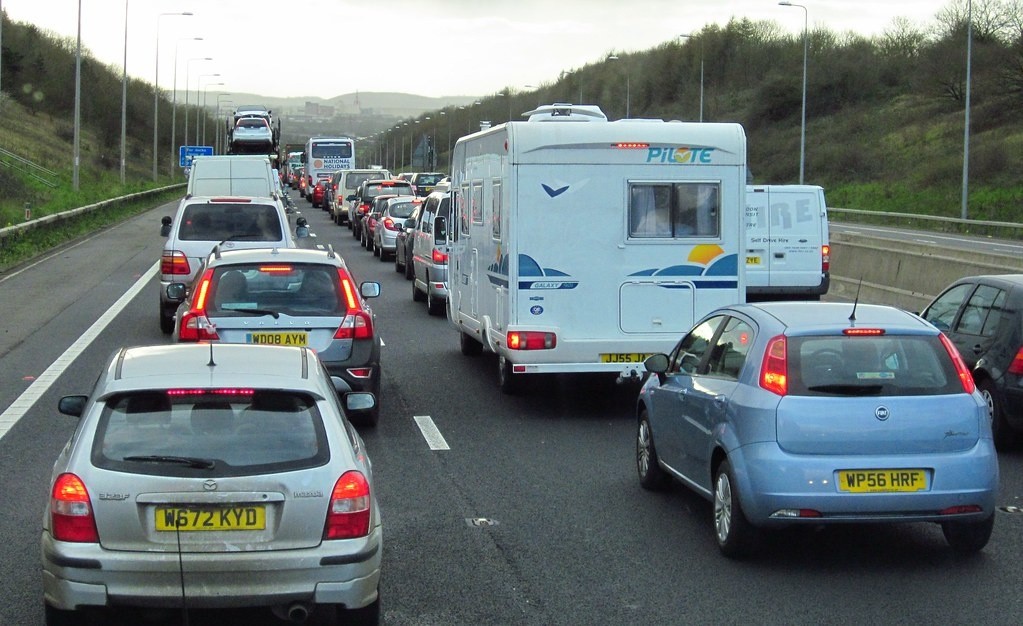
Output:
[432,103,748,394]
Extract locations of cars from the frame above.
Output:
[187,103,454,315]
[634,300,1001,557]
[38,344,384,626]
[912,273,1023,442]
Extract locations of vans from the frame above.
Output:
[745,183,832,302]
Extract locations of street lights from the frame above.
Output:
[778,0,809,185]
[609,55,630,118]
[426,116,435,172]
[380,131,389,170]
[387,127,396,176]
[565,70,584,104]
[525,84,541,108]
[184,57,213,177]
[170,37,205,181]
[497,94,512,122]
[439,111,451,177]
[152,10,195,183]
[395,125,404,173]
[414,120,425,172]
[458,103,470,135]
[215,93,232,155]
[202,82,225,146]
[475,101,487,121]
[403,122,412,172]
[679,34,704,122]
[196,72,222,147]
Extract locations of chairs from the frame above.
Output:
[117,391,310,444]
[215,270,250,311]
[294,269,337,310]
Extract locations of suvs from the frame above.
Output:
[165,242,382,428]
[158,192,309,334]
